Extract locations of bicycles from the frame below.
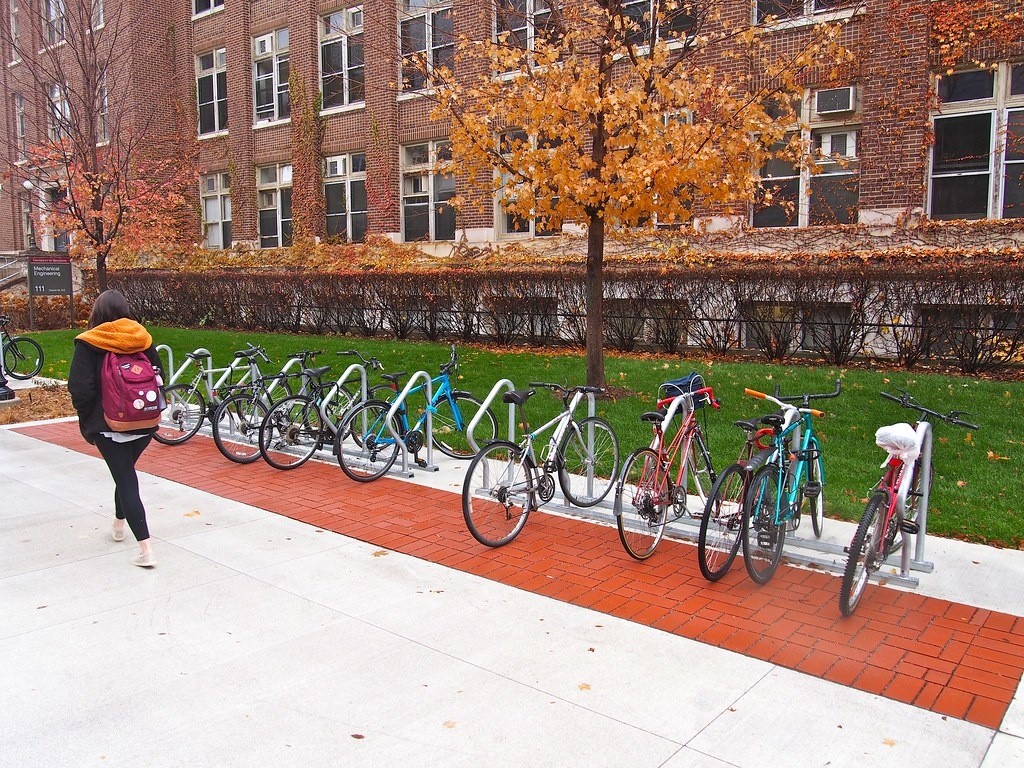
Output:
[462,380,620,547]
[839,387,978,615]
[333,343,498,482]
[0,315,44,380]
[211,345,354,464]
[258,350,408,470]
[613,387,720,560]
[697,376,843,585]
[152,342,277,445]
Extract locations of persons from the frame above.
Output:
[68,290,166,566]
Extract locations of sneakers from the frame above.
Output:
[132,552,156,567]
[110,522,125,541]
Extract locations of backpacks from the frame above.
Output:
[100,345,162,432]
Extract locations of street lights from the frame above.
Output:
[23,180,40,250]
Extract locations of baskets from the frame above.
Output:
[663,370,706,414]
